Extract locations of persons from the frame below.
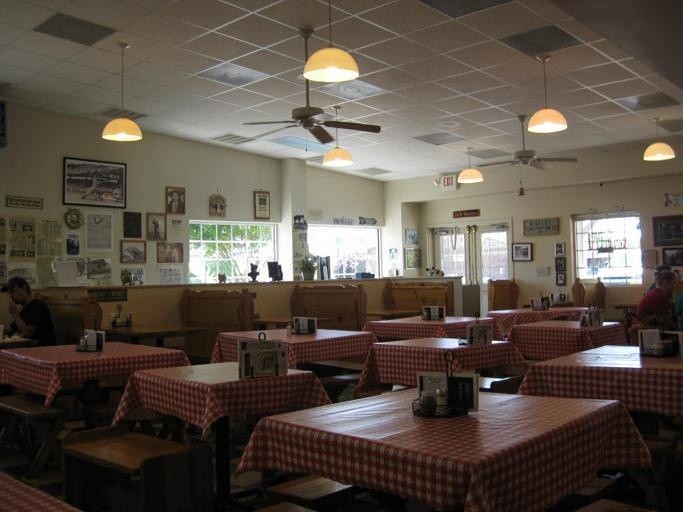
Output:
[635,264,683,335]
[167,189,184,214]
[1,276,58,347]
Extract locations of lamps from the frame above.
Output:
[323,103,353,167]
[525,53,570,134]
[304,0,363,83]
[643,117,676,162]
[458,148,483,184]
[102,37,142,141]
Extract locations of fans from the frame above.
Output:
[474,116,580,174]
[240,27,382,146]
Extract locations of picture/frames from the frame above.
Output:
[63,158,129,210]
[406,248,422,270]
[253,192,272,220]
[511,244,532,262]
[523,218,560,237]
[406,229,419,244]
[147,211,167,241]
[555,241,566,257]
[652,215,683,246]
[555,258,567,271]
[556,272,567,286]
[156,243,184,264]
[124,241,147,264]
[662,248,683,266]
[167,185,186,215]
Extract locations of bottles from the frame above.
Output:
[116,316,121,325]
[417,389,437,415]
[79,335,87,349]
[589,277,608,308]
[654,339,673,354]
[287,323,292,336]
[543,299,547,310]
[422,308,428,320]
[571,277,586,306]
[435,388,448,415]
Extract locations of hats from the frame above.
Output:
[0,275,27,293]
